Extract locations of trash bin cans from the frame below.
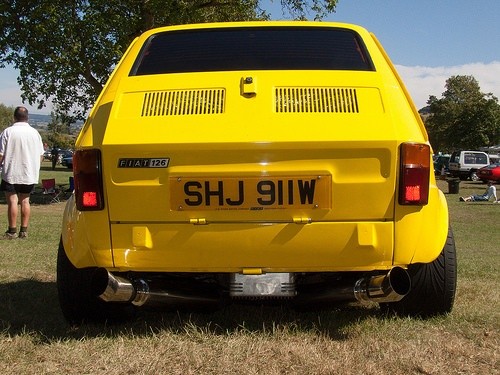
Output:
[447,177,460,194]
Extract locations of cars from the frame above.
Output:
[55,20,458,328]
[433,150,500,184]
[43,147,74,169]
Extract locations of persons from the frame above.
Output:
[459,180,497,202]
[0,106,45,239]
[51,145,60,170]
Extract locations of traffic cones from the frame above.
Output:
[436,165,448,180]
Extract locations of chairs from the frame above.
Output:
[41,177,75,205]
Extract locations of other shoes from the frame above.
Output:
[18,231,27,239]
[460,197,466,202]
[0,232,18,241]
